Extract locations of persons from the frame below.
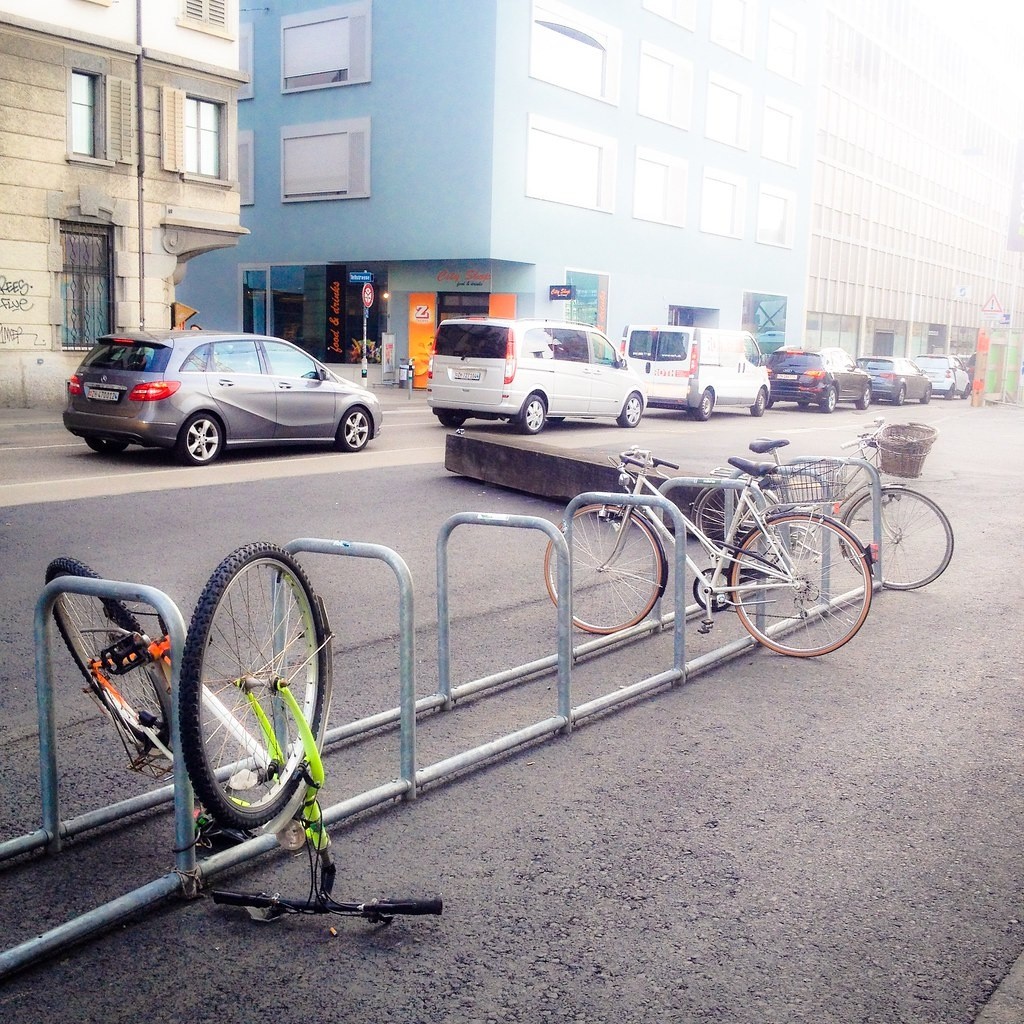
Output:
[966,352,977,382]
[190,324,203,330]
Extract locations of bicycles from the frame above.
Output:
[45,538,446,929]
[544,442,879,659]
[688,421,952,592]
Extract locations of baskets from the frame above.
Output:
[775,462,846,504]
[877,422,939,479]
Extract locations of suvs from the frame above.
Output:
[766,345,874,414]
[914,354,972,400]
[425,314,649,436]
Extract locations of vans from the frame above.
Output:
[619,322,770,420]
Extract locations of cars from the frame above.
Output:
[957,352,977,378]
[61,329,384,467]
[859,356,932,406]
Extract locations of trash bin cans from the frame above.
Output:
[396,363,414,389]
